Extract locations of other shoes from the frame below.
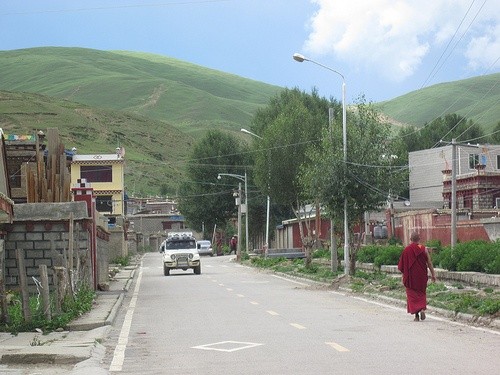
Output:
[413,317,419,321]
[421,312,425,320]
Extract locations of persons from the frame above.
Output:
[397,231,437,322]
[227,236,237,255]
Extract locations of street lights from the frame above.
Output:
[217,172,248,252]
[293,53,350,277]
[240,129,271,249]
[383,155,398,197]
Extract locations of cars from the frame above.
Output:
[196,241,213,256]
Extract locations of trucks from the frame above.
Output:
[163,234,201,276]
